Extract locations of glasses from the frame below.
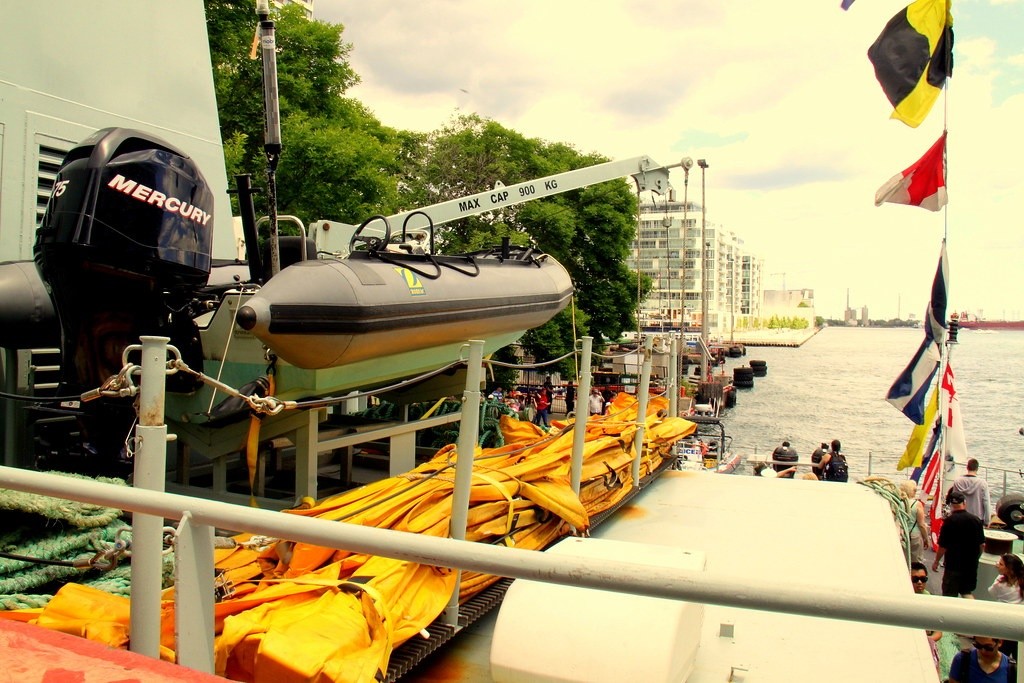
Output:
[912,575,928,584]
[972,640,998,651]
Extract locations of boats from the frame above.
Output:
[33,126,576,461]
[669,415,741,475]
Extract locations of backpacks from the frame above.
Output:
[828,452,848,482]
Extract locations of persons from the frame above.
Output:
[488,388,551,427]
[590,384,615,415]
[564,380,575,416]
[988,552,1024,662]
[544,376,554,414]
[899,480,929,562]
[952,458,990,527]
[949,636,1009,683]
[772,440,845,480]
[911,562,942,667]
[932,492,985,599]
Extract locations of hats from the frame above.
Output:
[950,492,966,505]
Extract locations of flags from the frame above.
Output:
[941,364,967,459]
[924,240,948,351]
[885,337,940,425]
[868,0,954,128]
[875,128,948,211]
[897,385,943,552]
